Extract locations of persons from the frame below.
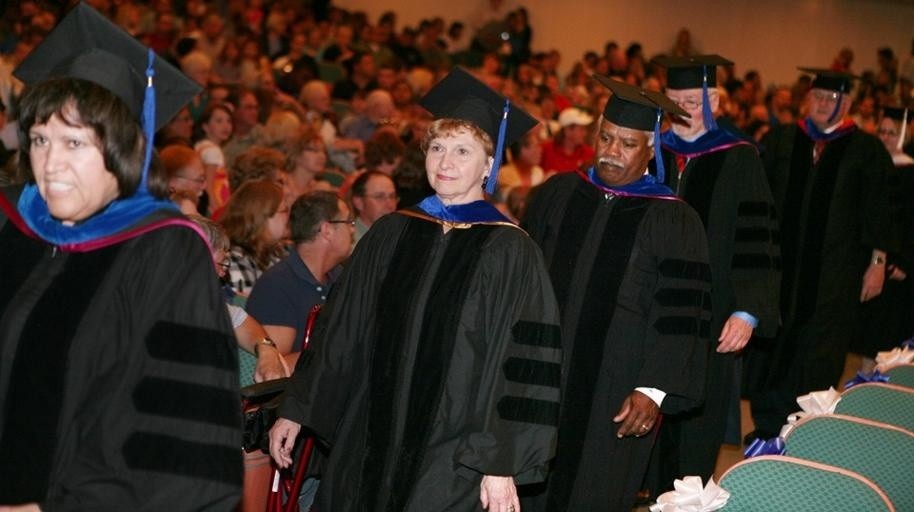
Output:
[157,144,207,216]
[566,26,698,131]
[155,103,194,153]
[1,0,246,512]
[85,0,562,109]
[647,54,783,491]
[268,67,564,512]
[538,107,595,177]
[339,127,407,201]
[713,63,813,145]
[278,131,331,203]
[0,0,57,187]
[215,181,290,298]
[349,170,398,254]
[242,189,356,384]
[850,100,914,360]
[297,79,336,143]
[834,42,914,145]
[744,65,895,449]
[514,73,711,512]
[222,90,266,175]
[183,211,286,383]
[347,89,394,141]
[191,102,233,218]
[496,132,544,204]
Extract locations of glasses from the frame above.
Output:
[671,99,703,110]
[173,172,207,185]
[304,146,327,153]
[239,105,259,110]
[329,216,356,227]
[216,256,232,271]
[276,205,292,214]
[366,194,401,202]
[178,115,192,122]
[812,92,840,102]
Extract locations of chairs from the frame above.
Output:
[833,384,914,433]
[716,456,896,512]
[780,416,913,511]
[881,366,914,390]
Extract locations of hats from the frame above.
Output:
[558,106,595,127]
[12,0,204,197]
[653,55,735,131]
[796,66,861,124]
[883,107,914,150]
[593,73,692,183]
[418,67,540,195]
